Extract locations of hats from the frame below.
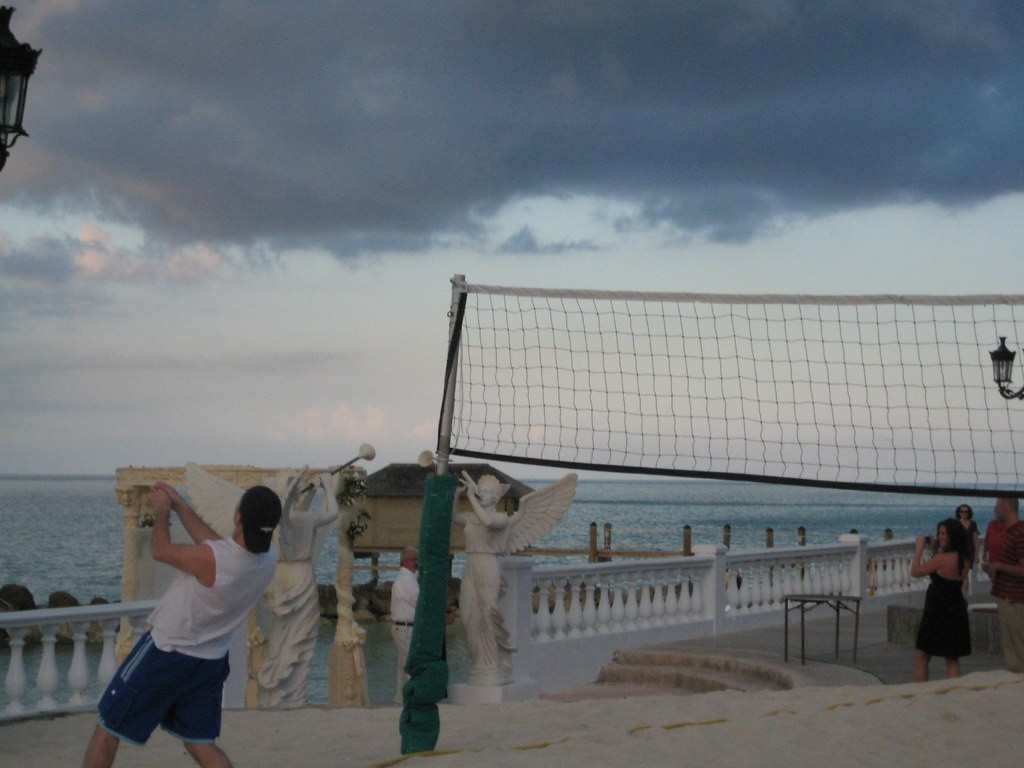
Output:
[238,486,282,554]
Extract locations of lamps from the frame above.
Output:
[990,336,1024,400]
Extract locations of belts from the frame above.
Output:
[395,621,414,627]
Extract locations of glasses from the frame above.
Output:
[959,510,969,514]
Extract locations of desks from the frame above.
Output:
[783,594,861,665]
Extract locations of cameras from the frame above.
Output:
[925,536,931,543]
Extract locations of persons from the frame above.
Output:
[981,506,1009,578]
[258,464,338,709]
[911,517,973,682]
[83,479,282,768]
[954,504,981,599]
[451,474,516,686]
[989,497,1024,673]
[392,550,419,704]
